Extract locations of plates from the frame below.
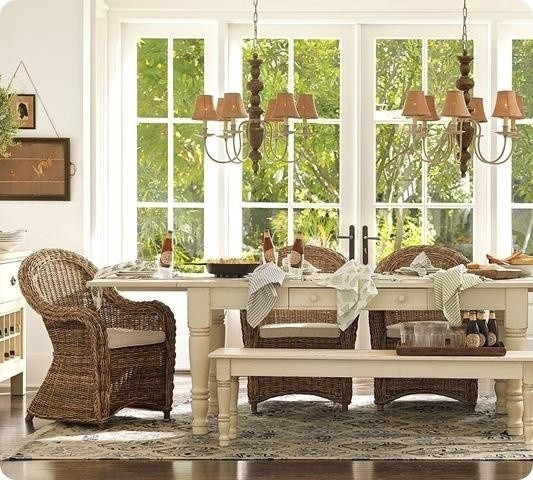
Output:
[396,270,436,275]
[116,273,154,276]
[118,271,155,273]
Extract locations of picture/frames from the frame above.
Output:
[0,137,72,201]
[8,94,35,128]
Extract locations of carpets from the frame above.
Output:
[1,390,533,461]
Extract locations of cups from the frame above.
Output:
[431,321,448,347]
[399,322,416,347]
[449,323,467,347]
[415,321,431,347]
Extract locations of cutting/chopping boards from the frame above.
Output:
[467,268,522,279]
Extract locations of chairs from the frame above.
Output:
[369,247,480,411]
[242,247,356,415]
[20,249,177,433]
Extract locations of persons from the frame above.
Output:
[18,103,28,119]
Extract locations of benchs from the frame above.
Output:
[209,346,532,445]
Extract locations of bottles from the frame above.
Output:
[462,310,469,331]
[264,229,275,262]
[477,312,488,346]
[291,231,304,267]
[160,231,172,268]
[488,310,499,346]
[467,311,479,348]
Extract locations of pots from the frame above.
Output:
[184,263,259,278]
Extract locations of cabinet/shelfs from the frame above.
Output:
[1,247,26,396]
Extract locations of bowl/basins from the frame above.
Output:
[0,235,23,253]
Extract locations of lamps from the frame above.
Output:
[192,2,320,176]
[400,0,524,176]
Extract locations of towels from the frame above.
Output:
[91,259,154,310]
[432,261,484,326]
[246,261,286,330]
[314,258,378,333]
[282,252,321,273]
[394,250,436,277]
